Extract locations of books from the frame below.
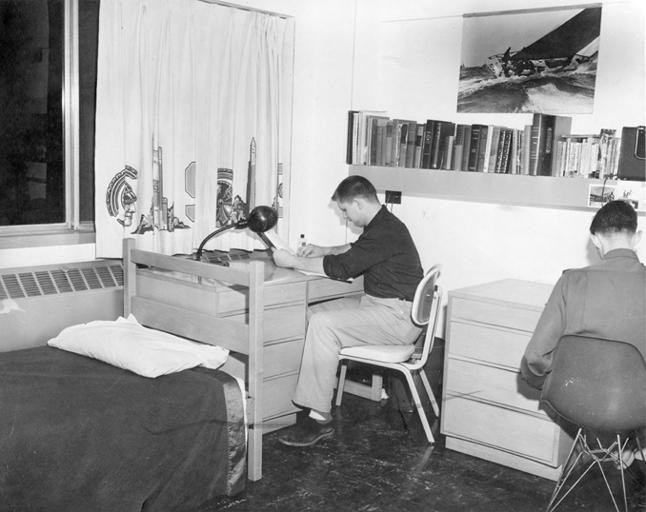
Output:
[295,270,353,283]
[345,108,622,181]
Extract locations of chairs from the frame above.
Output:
[537,335,646,510]
[338,262,441,446]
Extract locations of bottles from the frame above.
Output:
[297,233,307,250]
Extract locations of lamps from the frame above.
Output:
[196,206,278,284]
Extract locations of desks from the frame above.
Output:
[140,264,365,435]
[439,278,582,484]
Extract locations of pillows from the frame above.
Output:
[44,312,230,380]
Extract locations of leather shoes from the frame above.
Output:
[278,415,335,447]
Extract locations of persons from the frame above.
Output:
[516,200,646,509]
[272,175,424,446]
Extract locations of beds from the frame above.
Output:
[0,239,264,511]
